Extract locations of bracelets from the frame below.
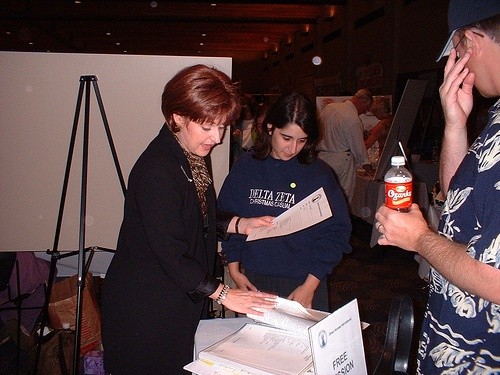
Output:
[215,284,230,305]
[235,217,242,234]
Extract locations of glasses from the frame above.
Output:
[455,31,483,58]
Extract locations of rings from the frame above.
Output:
[378,224,382,233]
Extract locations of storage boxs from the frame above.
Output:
[46,274,101,354]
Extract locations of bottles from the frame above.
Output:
[384,155,413,213]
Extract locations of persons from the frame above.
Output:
[373,0,500,375]
[255,101,274,135]
[231,102,260,151]
[102,63,277,375]
[215,90,353,319]
[316,89,378,254]
[364,96,399,167]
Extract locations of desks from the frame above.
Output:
[193,317,252,362]
[351,161,439,248]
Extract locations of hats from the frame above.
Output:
[435,0,500,61]
